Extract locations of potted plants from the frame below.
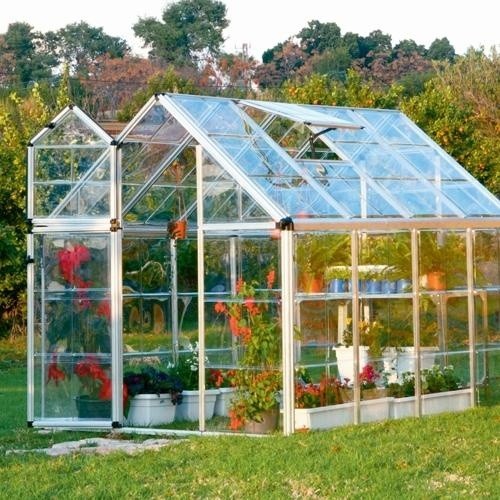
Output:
[391,367,473,419]
[392,311,440,375]
[392,234,462,293]
[394,270,412,293]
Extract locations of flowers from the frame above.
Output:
[54,247,391,435]
